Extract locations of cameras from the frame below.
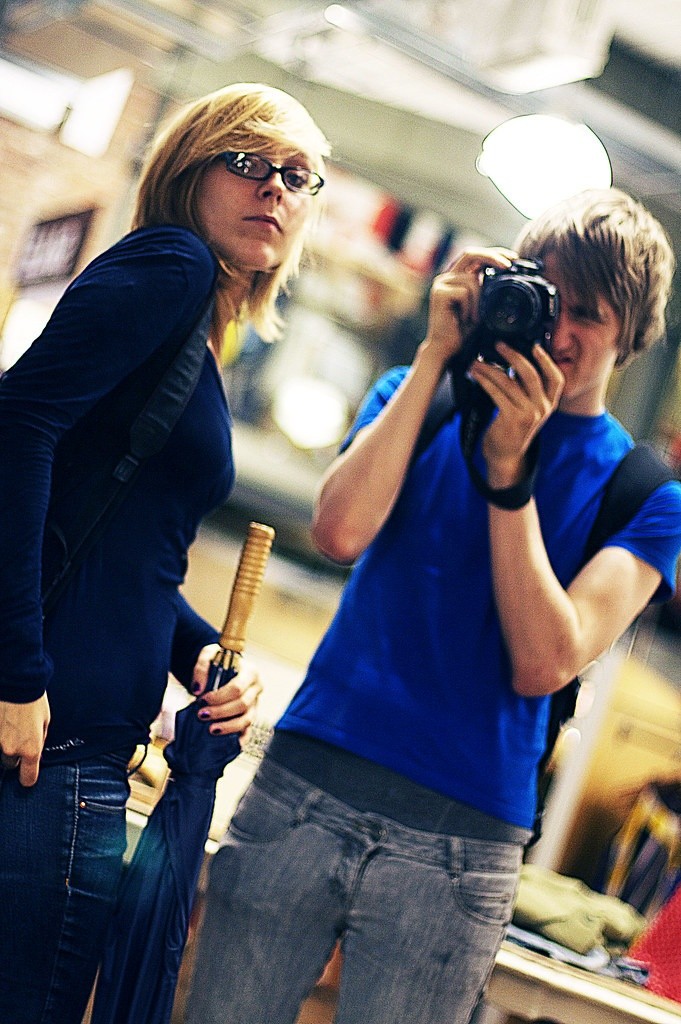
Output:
[469,257,559,358]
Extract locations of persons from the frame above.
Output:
[182,188,681,1024]
[0,82,331,1024]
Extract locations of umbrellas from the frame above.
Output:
[89,522,275,1024]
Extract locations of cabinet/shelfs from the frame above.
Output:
[475,881,680,1024]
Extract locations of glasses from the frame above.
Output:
[215,152,325,196]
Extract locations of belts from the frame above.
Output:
[104,744,136,764]
